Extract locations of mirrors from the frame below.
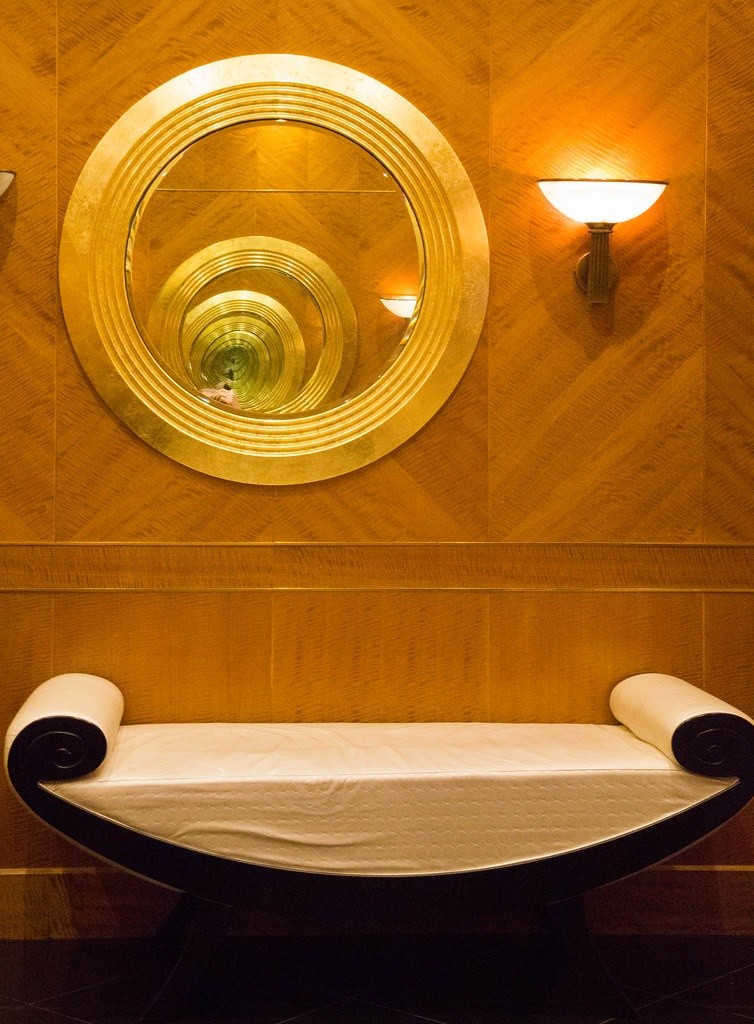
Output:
[142,232,359,420]
[55,53,491,483]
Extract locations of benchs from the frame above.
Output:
[3,672,754,1023]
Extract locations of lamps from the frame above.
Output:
[537,178,669,304]
